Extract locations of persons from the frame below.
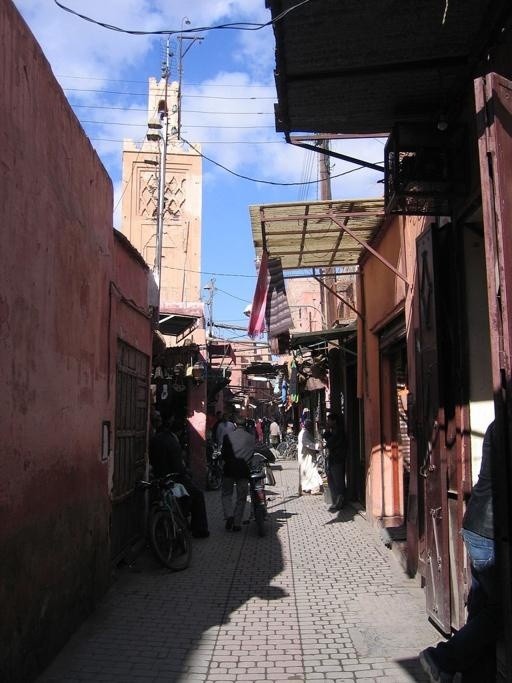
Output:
[159,414,175,431]
[322,413,346,509]
[296,419,326,495]
[419,420,498,683]
[149,420,209,538]
[221,416,275,531]
[210,411,283,453]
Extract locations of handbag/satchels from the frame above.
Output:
[261,460,275,485]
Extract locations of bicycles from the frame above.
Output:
[137,470,193,572]
[275,433,298,461]
[313,437,329,477]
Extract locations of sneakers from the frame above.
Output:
[225,516,234,528]
[419,645,453,682]
[233,526,242,530]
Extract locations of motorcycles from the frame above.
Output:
[206,438,223,490]
[247,457,276,537]
